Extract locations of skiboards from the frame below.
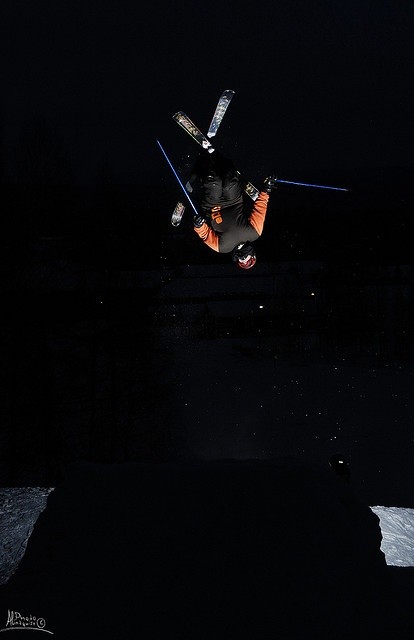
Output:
[171,89,260,227]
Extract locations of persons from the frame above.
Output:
[192,151,279,270]
